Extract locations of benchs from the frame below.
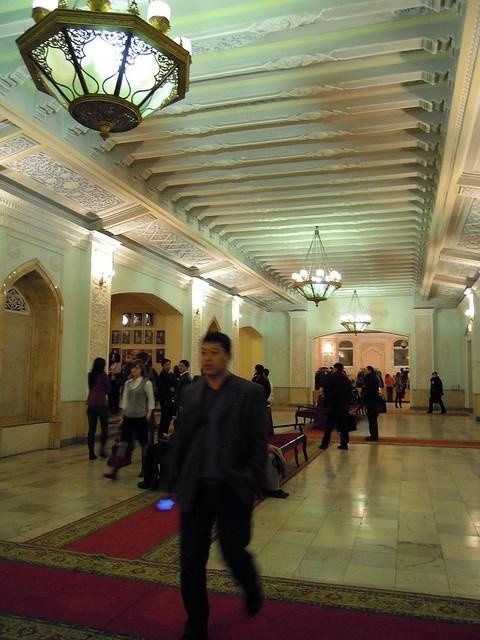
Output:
[266,406,308,477]
[294,396,366,429]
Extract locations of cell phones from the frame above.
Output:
[155,497,176,512]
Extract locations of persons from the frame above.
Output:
[426,371,447,415]
[315,363,409,450]
[112,313,164,344]
[83,351,294,501]
[174,330,267,639]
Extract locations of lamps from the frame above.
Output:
[15,1,191,141]
[93,267,115,286]
[339,290,371,336]
[401,341,407,348]
[292,226,343,308]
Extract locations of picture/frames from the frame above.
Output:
[110,313,165,364]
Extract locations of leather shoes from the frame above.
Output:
[135,478,156,491]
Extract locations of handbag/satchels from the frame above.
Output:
[106,441,133,468]
[368,395,387,414]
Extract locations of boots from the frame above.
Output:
[86,438,108,460]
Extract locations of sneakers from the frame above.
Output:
[102,473,117,480]
[318,442,349,450]
[265,488,291,499]
[243,579,265,618]
